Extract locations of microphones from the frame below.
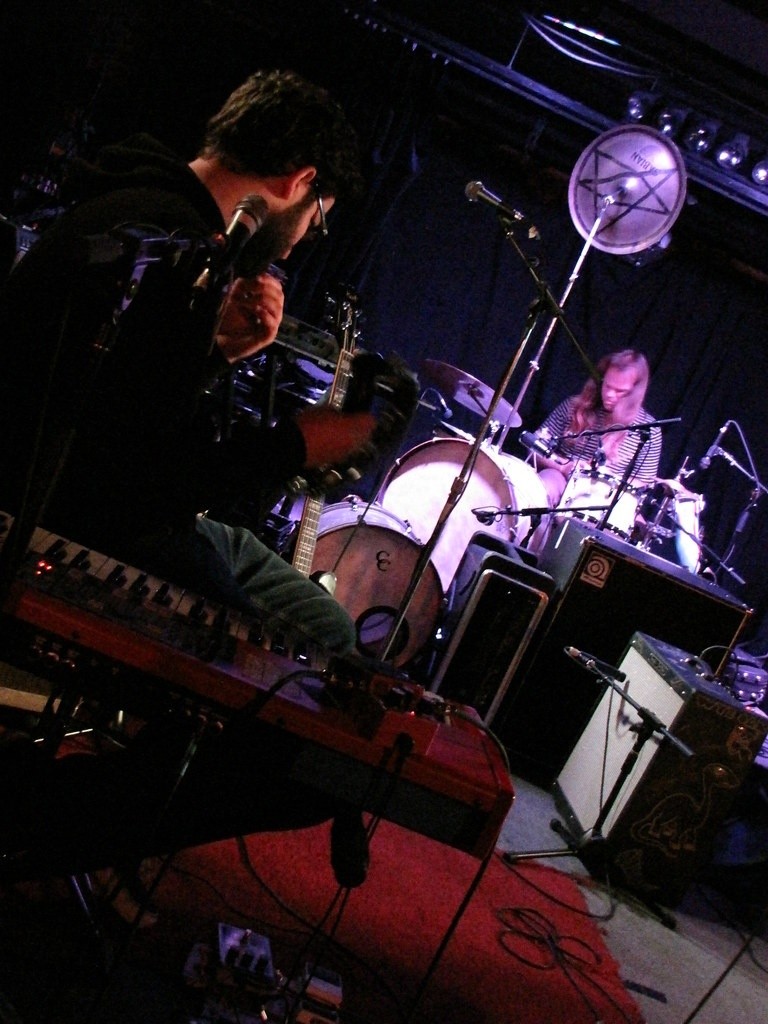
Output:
[563,647,626,682]
[701,423,730,469]
[471,509,495,526]
[520,432,561,464]
[433,391,454,419]
[191,191,269,311]
[466,180,538,238]
[598,438,607,467]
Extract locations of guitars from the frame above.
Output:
[290,287,364,600]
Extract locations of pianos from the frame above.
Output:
[0,505,513,853]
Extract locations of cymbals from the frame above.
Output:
[418,352,521,426]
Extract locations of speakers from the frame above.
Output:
[488,517,768,884]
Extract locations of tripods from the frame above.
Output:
[502,653,701,930]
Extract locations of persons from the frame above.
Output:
[3,66,361,885]
[529,348,662,554]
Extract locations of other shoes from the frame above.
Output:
[0,730,53,890]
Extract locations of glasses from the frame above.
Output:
[296,183,328,243]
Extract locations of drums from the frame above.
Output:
[551,463,646,544]
[635,491,705,577]
[286,502,444,679]
[376,436,549,601]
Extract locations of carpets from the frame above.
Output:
[134,812,646,1024]
